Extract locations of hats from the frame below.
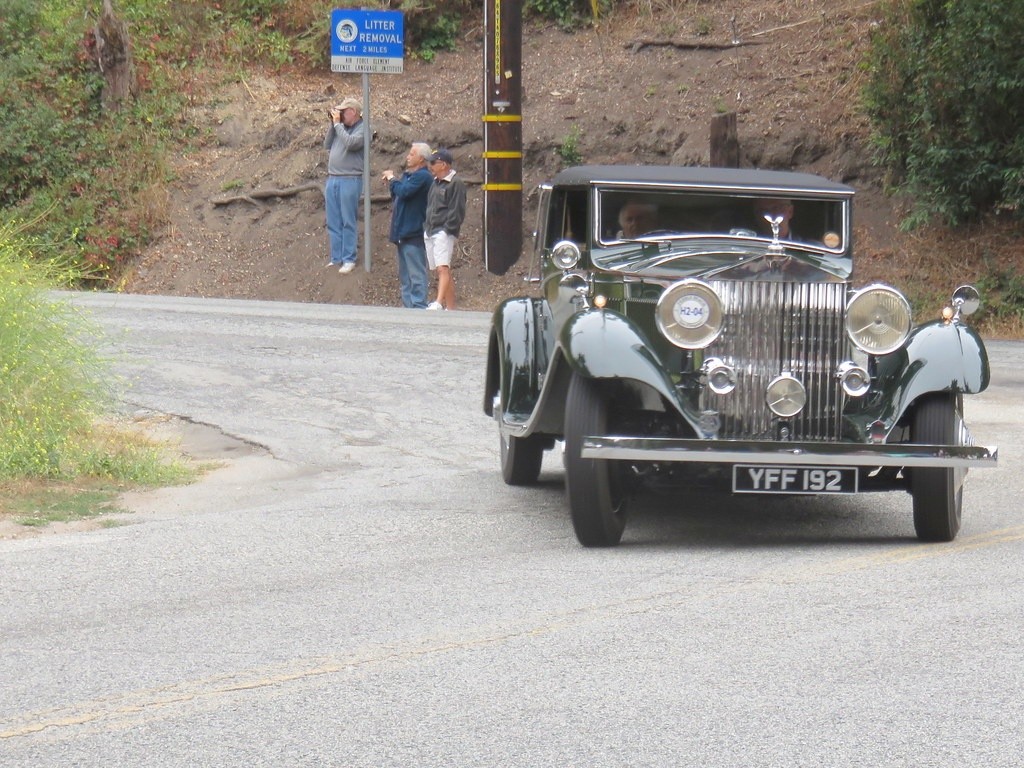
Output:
[424,149,453,164]
[335,98,363,112]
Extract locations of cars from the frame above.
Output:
[484,165,998,548]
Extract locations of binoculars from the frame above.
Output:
[328,110,341,118]
[382,175,386,180]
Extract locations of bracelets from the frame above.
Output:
[388,175,395,181]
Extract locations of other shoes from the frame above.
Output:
[339,263,355,273]
[426,302,447,311]
[326,263,334,267]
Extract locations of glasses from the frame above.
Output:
[756,204,790,216]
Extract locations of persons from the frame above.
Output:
[422,148,467,312]
[614,200,659,240]
[324,98,364,274]
[754,200,824,249]
[380,142,433,309]
